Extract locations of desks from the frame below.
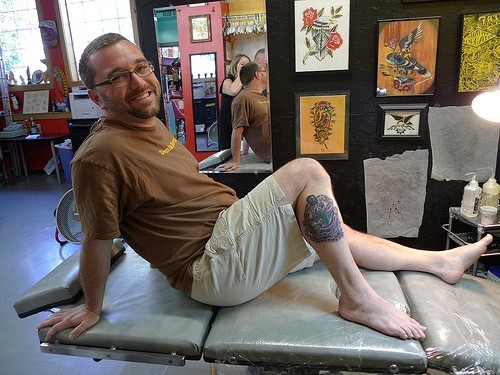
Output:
[0,132,69,183]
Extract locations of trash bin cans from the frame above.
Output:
[55,138,73,184]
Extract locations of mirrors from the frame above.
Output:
[152,0,274,174]
[189,52,220,153]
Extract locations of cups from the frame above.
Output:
[480,206,497,224]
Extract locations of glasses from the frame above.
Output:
[90,60,155,90]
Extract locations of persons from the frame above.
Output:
[254,48,268,98]
[218,54,252,151]
[37,32,495,341]
[217,61,272,171]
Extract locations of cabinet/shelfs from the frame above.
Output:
[440,205,500,280]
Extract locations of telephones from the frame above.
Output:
[4,122,24,131]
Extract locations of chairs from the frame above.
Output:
[169,90,187,144]
[12,237,500,375]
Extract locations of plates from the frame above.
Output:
[31,70,44,84]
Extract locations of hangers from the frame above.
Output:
[221,14,267,36]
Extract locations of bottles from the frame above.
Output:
[22,120,30,135]
[29,117,37,134]
[461,179,481,217]
[482,178,500,215]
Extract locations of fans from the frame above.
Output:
[207,122,219,147]
[54,189,88,244]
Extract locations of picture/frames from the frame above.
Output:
[293,89,351,161]
[376,102,431,142]
[289,0,355,76]
[188,14,212,44]
[453,11,500,95]
[375,16,441,97]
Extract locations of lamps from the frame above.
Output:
[472,76,500,123]
